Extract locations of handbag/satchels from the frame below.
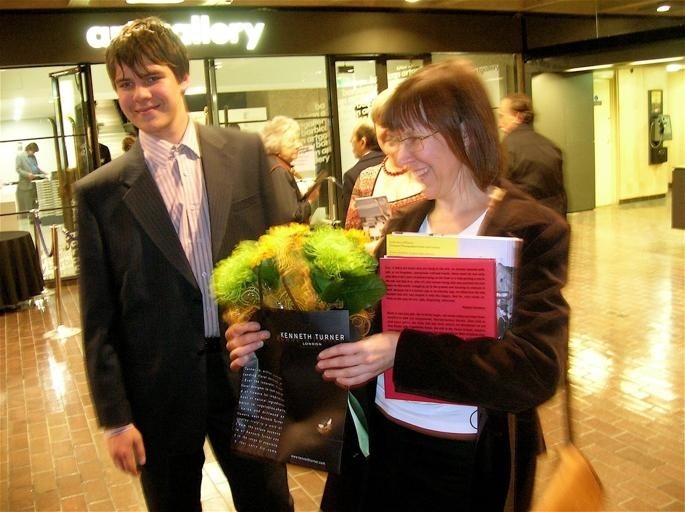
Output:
[528,436,609,512]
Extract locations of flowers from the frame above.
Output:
[210,217,384,344]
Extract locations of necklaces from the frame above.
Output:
[382,155,408,176]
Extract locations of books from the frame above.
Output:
[355,195,392,238]
[374,229,525,445]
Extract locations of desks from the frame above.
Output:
[0,231,44,311]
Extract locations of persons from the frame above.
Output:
[97,142,112,167]
[121,136,136,151]
[494,93,568,221]
[67,15,294,511]
[345,87,430,252]
[14,142,48,221]
[338,124,386,229]
[225,65,573,512]
[255,114,321,234]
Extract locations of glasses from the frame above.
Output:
[395,128,440,154]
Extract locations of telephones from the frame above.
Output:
[653,114,672,143]
